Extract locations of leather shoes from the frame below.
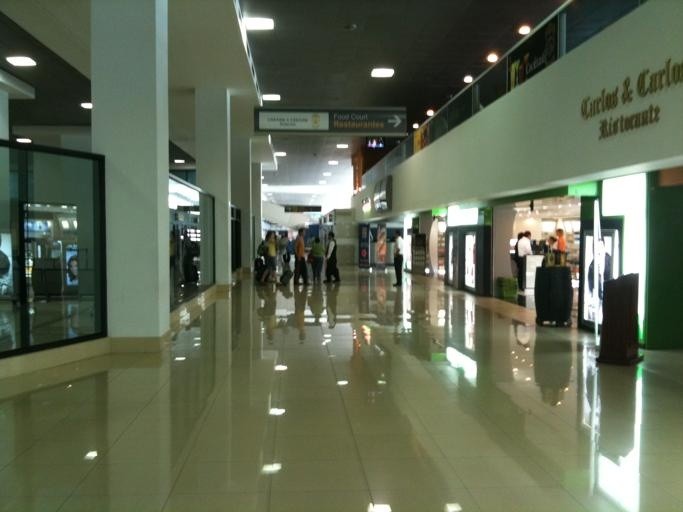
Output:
[392,281,403,286]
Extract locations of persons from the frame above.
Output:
[391,229,403,286]
[67,255,79,281]
[260,228,341,285]
[515,230,532,291]
[169,229,200,283]
[549,228,566,265]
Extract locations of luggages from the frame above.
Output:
[536,266,572,326]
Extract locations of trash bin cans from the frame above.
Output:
[254,258,263,270]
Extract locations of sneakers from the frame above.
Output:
[258,279,342,284]
